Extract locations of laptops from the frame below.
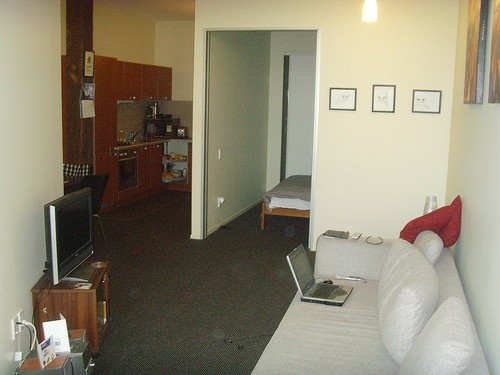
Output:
[286,244,353,307]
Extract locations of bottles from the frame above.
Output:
[423,196,438,215]
[182,168,187,177]
[119,129,125,142]
[151,101,159,119]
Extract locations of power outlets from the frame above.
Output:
[11,309,26,340]
[218,197,222,208]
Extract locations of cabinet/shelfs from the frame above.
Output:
[92,55,192,213]
[32,260,109,357]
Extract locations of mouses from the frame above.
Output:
[322,280,333,284]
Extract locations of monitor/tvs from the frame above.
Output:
[43,187,94,285]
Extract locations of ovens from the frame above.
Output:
[118,150,139,192]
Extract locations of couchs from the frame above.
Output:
[250,232,489,375]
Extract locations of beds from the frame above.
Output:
[261,174,312,230]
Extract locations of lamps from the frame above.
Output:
[362,0,377,22]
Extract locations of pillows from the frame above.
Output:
[414,229,445,265]
[438,195,461,247]
[379,239,438,365]
[400,204,455,243]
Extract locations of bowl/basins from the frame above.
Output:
[172,172,181,178]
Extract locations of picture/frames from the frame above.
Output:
[412,90,442,113]
[329,88,356,111]
[372,84,396,112]
